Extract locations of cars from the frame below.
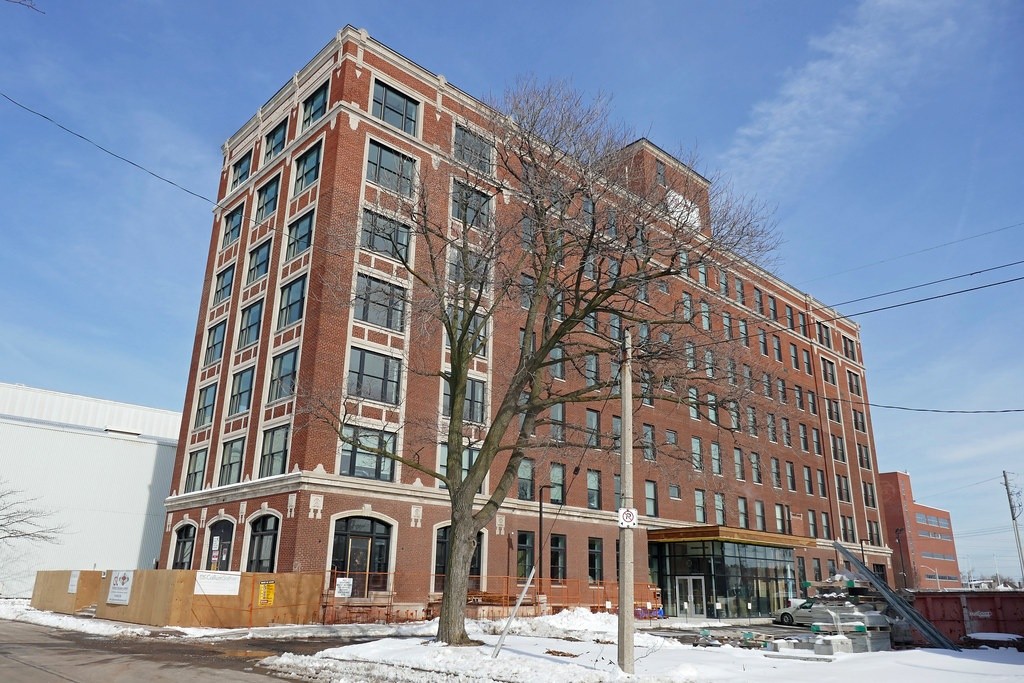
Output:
[776,600,859,627]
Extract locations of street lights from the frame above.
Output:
[895,527,909,588]
[860,538,872,565]
